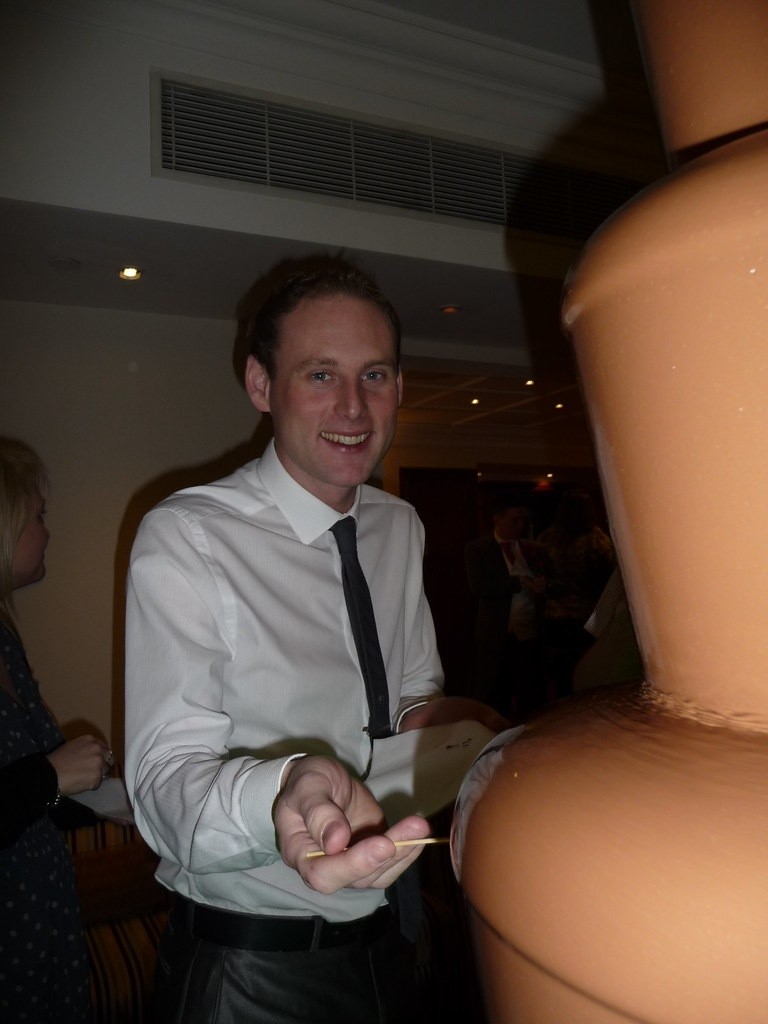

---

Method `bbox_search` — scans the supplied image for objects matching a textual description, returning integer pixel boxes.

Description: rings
[106,751,113,763]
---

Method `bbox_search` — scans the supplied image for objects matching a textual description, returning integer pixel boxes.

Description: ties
[331,516,395,739]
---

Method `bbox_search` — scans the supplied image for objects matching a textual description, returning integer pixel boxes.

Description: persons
[0,436,116,1024]
[122,252,515,1024]
[463,502,644,723]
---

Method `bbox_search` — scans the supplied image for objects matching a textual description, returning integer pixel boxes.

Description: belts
[192,896,469,952]
